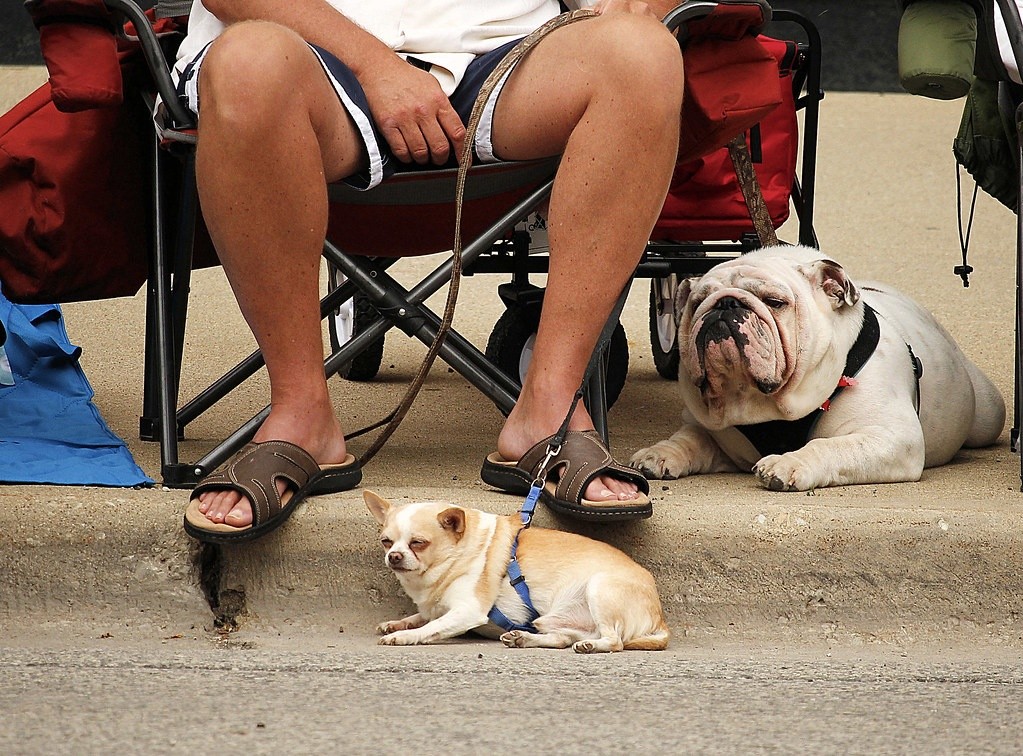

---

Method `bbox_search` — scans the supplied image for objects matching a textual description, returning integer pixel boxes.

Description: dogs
[629,245,1006,491]
[363,490,670,652]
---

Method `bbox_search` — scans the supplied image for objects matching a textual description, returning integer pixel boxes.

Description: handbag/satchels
[649,0,798,240]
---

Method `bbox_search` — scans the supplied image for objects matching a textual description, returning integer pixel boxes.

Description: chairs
[0,1,616,488]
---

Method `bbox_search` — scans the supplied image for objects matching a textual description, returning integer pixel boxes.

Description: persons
[172,0,684,546]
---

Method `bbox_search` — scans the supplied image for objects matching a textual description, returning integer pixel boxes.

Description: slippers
[480,429,652,522]
[184,440,363,544]
